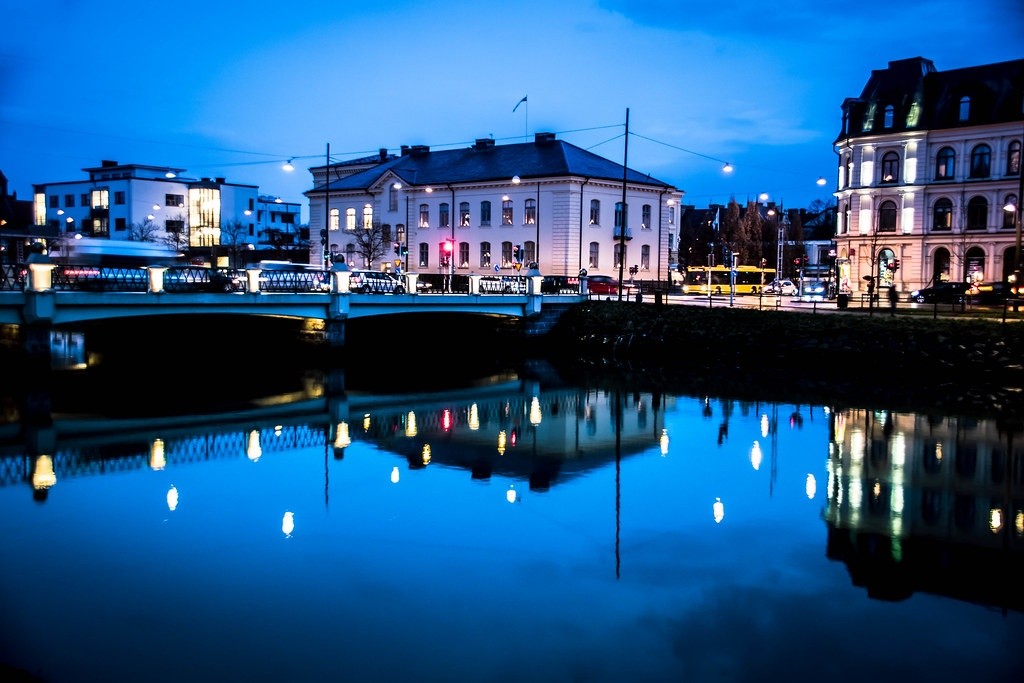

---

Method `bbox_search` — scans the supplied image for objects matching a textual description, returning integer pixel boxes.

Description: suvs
[350,269,406,294]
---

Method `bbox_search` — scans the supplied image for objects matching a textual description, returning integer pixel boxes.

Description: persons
[822,279,828,289]
[888,284,899,317]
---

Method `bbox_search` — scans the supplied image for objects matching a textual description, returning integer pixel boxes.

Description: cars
[588,275,639,297]
[761,279,797,295]
[806,282,833,296]
[910,281,1012,307]
[481,273,530,293]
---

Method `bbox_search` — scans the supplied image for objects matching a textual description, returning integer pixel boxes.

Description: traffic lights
[795,258,802,271]
[635,265,638,273]
[325,250,329,260]
[513,244,520,259]
[394,244,400,254]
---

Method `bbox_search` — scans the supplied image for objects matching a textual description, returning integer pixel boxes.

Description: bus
[684,266,775,295]
[55,240,326,296]
